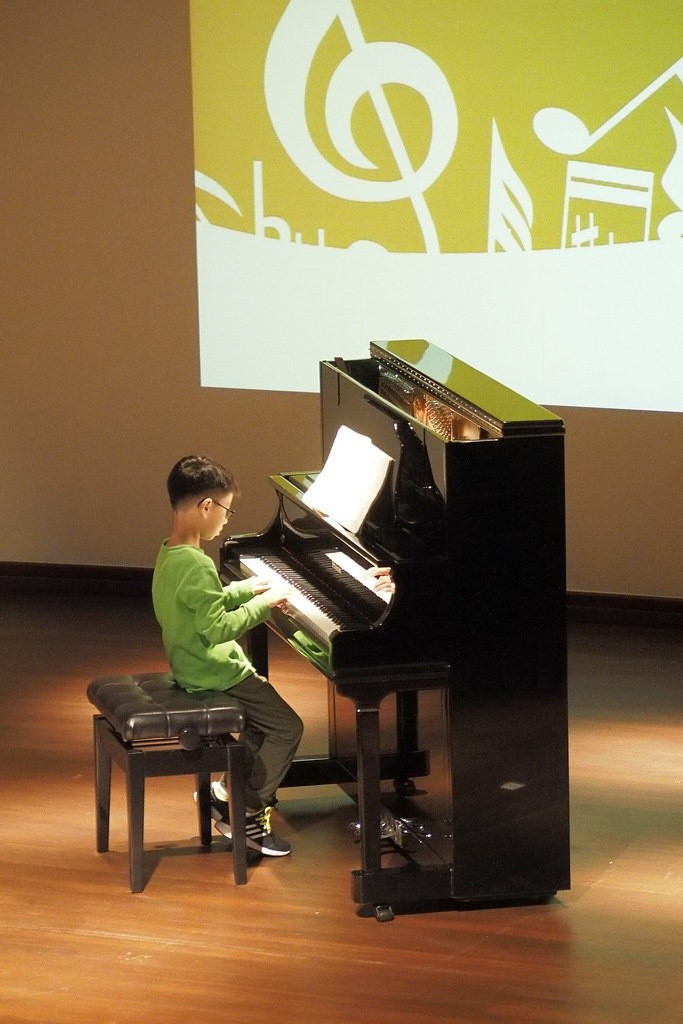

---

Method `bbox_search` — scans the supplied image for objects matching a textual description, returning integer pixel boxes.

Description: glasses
[196,496,235,520]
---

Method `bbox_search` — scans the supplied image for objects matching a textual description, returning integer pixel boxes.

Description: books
[302,425,396,533]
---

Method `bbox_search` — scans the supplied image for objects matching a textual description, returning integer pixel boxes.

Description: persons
[363,567,393,592]
[152,455,304,855]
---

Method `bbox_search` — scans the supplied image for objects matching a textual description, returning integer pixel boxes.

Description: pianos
[211,335,573,923]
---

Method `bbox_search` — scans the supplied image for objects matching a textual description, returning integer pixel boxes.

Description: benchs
[88,670,248,891]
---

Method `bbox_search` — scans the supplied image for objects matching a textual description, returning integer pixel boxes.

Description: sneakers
[193,774,229,821]
[214,799,291,857]
